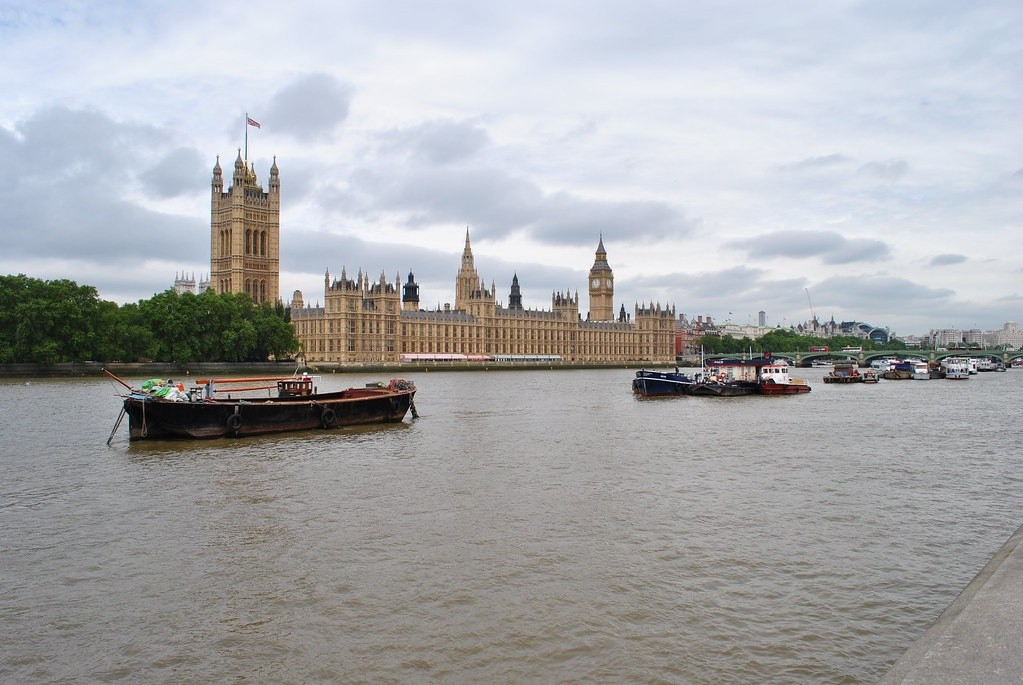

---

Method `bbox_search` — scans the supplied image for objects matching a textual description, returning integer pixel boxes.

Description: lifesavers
[322,408,335,424]
[227,414,243,430]
[721,372,726,378]
[392,401,400,414]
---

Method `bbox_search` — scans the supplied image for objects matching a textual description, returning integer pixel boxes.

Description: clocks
[592,278,600,289]
[606,279,613,289]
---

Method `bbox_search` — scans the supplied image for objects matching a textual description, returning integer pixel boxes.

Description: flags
[248,117,260,129]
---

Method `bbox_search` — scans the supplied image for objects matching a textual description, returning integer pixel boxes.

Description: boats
[874,356,931,380]
[632,367,694,395]
[101,366,416,446]
[939,356,1023,380]
[823,363,879,384]
[693,343,812,397]
